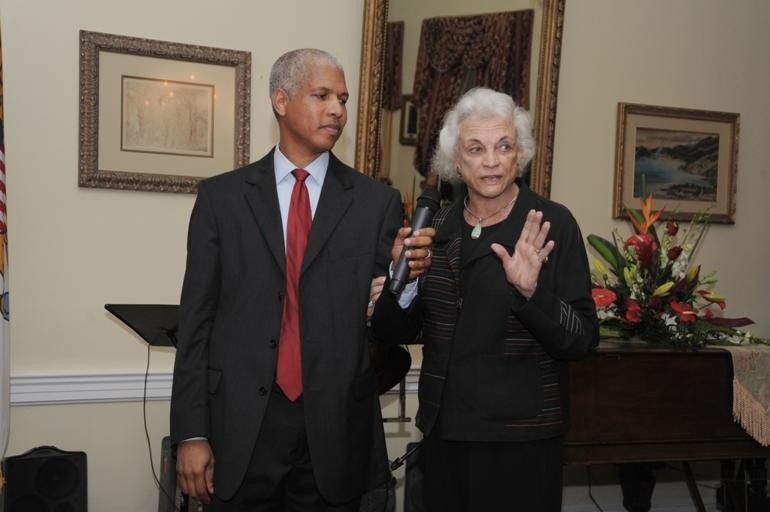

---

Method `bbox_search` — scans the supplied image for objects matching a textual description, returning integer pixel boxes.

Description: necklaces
[463,183,519,240]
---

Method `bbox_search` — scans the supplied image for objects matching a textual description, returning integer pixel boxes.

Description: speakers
[0,445,88,512]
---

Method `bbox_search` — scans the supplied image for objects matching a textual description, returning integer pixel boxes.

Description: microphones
[389,186,441,296]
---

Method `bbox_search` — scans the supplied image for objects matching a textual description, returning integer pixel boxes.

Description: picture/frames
[78,30,252,191]
[399,94,421,145]
[612,102,740,225]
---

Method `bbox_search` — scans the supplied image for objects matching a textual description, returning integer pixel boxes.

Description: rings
[368,299,376,307]
[425,249,430,259]
[534,246,543,253]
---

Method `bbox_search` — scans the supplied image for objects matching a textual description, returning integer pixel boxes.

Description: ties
[276,168,314,401]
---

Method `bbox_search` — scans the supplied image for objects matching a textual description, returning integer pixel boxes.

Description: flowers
[582,174,770,352]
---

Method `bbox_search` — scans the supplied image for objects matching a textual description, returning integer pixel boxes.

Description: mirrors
[354,1,564,227]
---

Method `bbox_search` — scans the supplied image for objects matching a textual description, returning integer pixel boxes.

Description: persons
[169,49,436,512]
[364,88,601,512]
